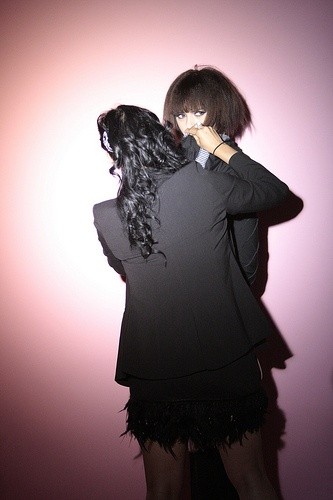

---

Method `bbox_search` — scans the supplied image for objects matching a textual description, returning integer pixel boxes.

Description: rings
[195,122,202,128]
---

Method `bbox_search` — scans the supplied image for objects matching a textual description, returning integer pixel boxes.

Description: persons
[163,64,261,500]
[93,104,294,500]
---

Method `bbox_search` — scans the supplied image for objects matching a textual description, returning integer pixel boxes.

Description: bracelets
[212,141,225,155]
